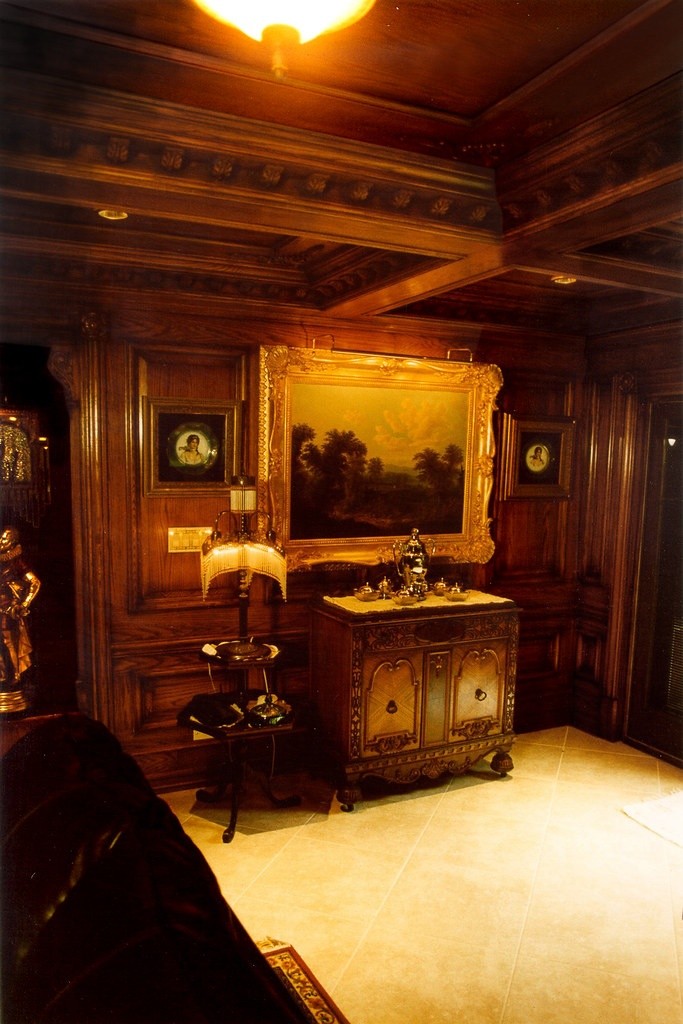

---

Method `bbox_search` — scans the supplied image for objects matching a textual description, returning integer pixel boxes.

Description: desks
[176,688,306,843]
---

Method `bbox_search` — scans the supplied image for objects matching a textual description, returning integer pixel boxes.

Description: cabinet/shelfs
[310,584,523,811]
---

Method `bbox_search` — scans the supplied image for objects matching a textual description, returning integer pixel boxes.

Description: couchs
[0,715,312,1024]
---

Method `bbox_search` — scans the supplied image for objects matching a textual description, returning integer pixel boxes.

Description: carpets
[255,934,354,1024]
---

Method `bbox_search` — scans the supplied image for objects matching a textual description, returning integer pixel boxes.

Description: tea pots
[443,583,470,601]
[408,583,428,600]
[390,585,417,604]
[430,578,452,596]
[355,583,380,602]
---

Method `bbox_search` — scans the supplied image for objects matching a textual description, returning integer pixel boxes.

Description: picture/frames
[140,393,249,499]
[258,342,506,574]
[498,410,579,503]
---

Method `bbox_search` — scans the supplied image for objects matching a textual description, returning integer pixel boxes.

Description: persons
[530,447,545,467]
[0,525,40,686]
[179,435,205,463]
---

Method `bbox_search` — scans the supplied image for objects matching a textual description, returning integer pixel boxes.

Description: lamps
[201,473,288,662]
[97,207,132,222]
[549,274,577,285]
